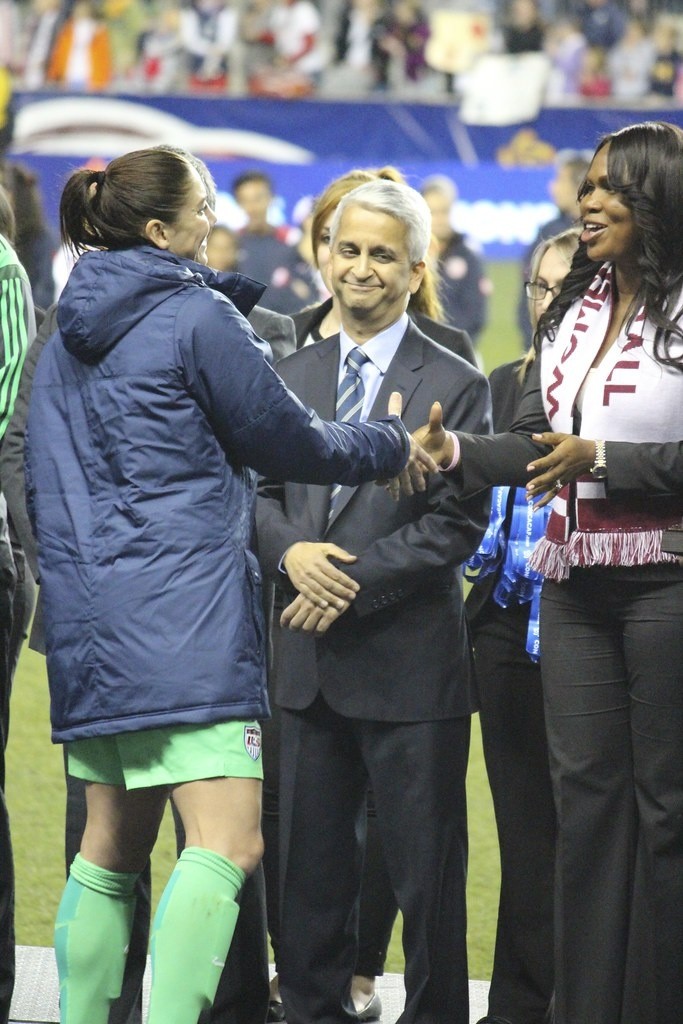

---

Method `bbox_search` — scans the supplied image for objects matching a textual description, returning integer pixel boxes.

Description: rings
[556,478,563,490]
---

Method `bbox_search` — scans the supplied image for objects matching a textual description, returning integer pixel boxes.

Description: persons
[0,147,277,1024]
[3,143,440,1023]
[419,172,487,346]
[514,147,599,354]
[0,0,683,320]
[461,225,593,1024]
[243,163,493,1023]
[246,176,481,1019]
[395,122,683,1024]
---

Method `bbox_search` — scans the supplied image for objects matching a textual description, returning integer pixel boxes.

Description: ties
[328,347,368,521]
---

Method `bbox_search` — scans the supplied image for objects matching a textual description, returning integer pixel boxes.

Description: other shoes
[357,993,381,1020]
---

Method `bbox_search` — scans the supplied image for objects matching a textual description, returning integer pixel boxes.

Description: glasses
[523,282,562,300]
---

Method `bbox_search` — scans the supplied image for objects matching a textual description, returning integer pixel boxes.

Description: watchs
[589,439,607,482]
